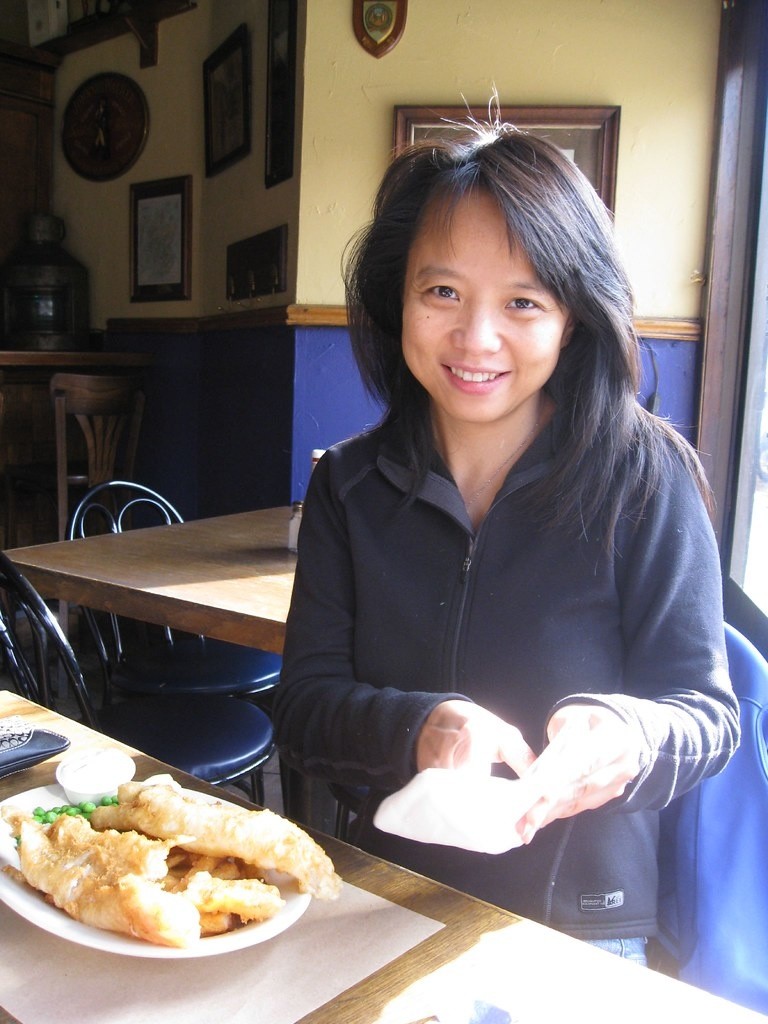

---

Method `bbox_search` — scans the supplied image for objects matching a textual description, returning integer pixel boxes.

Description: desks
[2,502,303,657]
[0,350,162,464]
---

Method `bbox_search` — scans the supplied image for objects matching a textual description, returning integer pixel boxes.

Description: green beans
[30,793,118,825]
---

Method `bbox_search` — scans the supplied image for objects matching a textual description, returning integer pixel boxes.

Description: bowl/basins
[55,747,137,808]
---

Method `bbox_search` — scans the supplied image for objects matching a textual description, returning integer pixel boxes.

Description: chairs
[335,576,768,1019]
[63,479,290,816]
[0,552,277,808]
[4,371,150,646]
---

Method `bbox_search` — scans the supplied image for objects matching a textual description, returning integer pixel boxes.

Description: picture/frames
[265,0,295,191]
[204,23,252,180]
[393,102,623,219]
[129,174,193,301]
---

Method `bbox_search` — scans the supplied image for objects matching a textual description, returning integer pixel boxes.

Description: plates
[0,784,312,959]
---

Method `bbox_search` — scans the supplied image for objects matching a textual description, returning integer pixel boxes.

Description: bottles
[310,449,327,480]
[288,501,303,553]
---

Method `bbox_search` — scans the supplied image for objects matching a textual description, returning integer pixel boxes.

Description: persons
[275,134,742,969]
[88,93,110,160]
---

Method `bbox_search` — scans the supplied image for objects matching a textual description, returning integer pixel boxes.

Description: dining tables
[0,691,768,1024]
